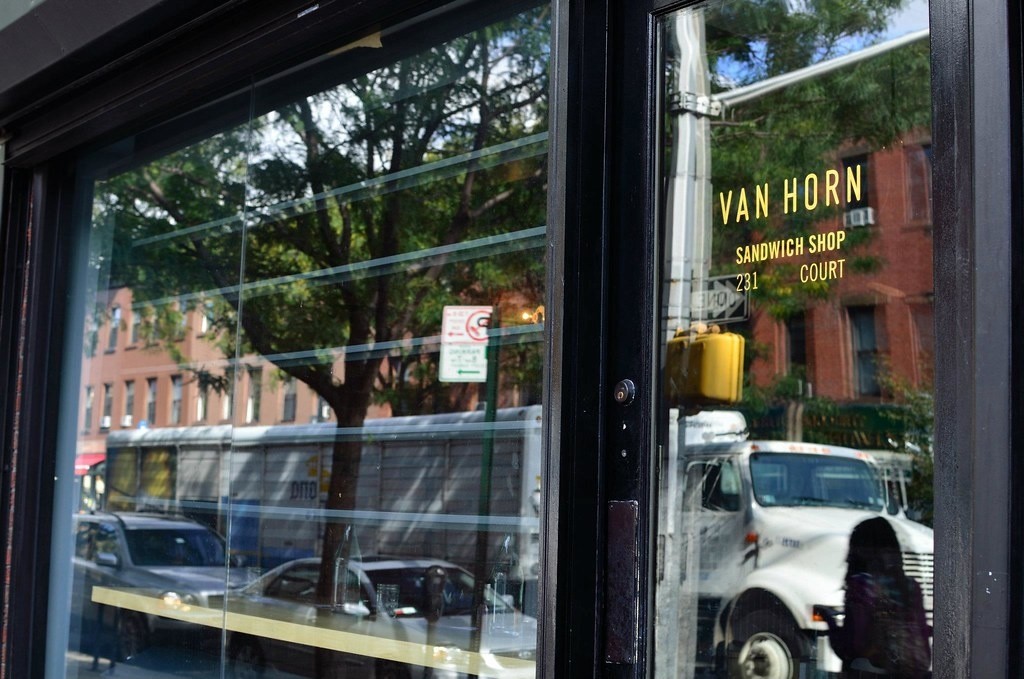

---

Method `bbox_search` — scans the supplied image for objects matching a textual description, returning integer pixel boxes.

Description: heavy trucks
[80,404,934,679]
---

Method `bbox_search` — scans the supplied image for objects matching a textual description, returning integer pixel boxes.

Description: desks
[91,585,537,679]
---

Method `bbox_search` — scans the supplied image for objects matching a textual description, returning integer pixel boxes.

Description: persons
[811,515,932,678]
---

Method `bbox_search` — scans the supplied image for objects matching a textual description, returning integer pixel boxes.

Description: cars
[219,554,539,679]
[70,510,314,664]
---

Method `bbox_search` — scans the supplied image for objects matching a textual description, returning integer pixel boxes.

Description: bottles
[489,537,524,636]
[331,525,362,613]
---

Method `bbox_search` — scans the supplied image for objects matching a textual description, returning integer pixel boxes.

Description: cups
[377,584,398,620]
